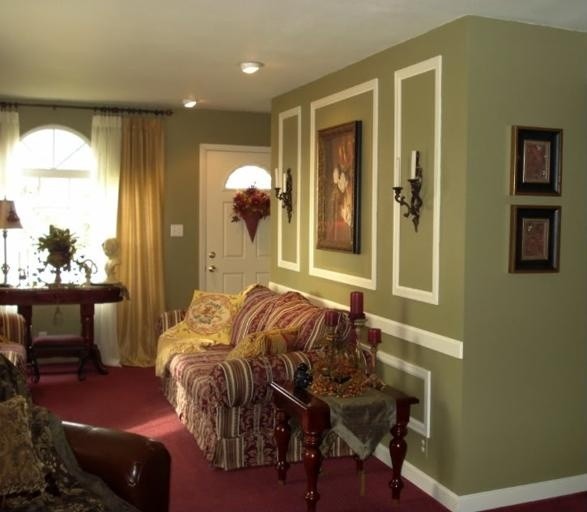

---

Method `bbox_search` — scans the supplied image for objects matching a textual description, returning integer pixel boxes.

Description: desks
[1,283,123,376]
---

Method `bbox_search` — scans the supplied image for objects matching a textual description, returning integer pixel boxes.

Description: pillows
[178,289,245,347]
[1,393,46,499]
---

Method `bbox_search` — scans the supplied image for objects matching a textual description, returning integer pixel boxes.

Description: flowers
[229,182,271,224]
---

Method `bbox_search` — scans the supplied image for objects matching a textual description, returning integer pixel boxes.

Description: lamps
[0,196,24,287]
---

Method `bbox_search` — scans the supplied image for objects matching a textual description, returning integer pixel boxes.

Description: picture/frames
[507,124,563,275]
[315,120,362,255]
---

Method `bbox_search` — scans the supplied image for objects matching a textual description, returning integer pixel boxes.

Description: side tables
[268,376,420,512]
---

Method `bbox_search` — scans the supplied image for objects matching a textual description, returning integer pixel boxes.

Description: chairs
[62,420,171,511]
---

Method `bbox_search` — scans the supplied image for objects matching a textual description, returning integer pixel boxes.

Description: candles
[324,291,381,345]
[395,150,417,188]
[275,168,287,193]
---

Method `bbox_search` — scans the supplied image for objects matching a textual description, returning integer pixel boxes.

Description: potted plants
[33,224,80,271]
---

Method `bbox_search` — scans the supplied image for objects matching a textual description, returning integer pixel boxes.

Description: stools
[29,334,91,383]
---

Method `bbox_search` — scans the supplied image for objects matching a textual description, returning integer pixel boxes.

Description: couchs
[154,283,359,471]
[0,309,27,374]
